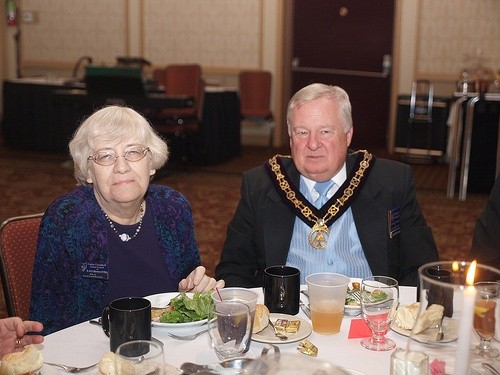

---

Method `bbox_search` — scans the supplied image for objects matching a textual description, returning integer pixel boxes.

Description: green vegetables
[345,287,387,305]
[160,288,215,323]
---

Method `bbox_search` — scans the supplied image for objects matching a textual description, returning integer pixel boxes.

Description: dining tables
[35,285,500,375]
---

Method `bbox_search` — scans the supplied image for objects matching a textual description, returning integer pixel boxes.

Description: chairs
[84,75,139,110]
[238,70,275,149]
[151,64,205,178]
[0,213,44,320]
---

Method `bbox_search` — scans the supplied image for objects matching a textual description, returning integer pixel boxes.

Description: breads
[98,351,137,375]
[234,304,270,333]
[0,345,44,375]
[396,302,444,333]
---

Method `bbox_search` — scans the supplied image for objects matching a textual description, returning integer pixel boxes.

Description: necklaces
[100,205,143,242]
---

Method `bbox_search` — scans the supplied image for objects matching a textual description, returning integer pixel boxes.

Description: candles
[453,260,478,375]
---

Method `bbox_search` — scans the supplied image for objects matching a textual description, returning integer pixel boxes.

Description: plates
[250,313,312,344]
[390,324,458,344]
[143,291,196,309]
[344,277,393,316]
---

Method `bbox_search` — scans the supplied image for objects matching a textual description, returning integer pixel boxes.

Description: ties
[313,180,334,210]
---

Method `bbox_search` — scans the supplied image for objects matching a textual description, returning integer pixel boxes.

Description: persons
[216,83,441,309]
[462,174,500,287]
[0,317,44,375]
[27,105,226,339]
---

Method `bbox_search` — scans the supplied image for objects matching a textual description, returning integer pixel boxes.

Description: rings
[16,338,21,348]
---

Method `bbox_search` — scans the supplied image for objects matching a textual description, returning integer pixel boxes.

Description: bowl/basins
[418,260,500,322]
[151,311,217,340]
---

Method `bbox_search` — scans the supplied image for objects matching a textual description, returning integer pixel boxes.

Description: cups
[361,276,399,351]
[207,302,256,357]
[306,272,351,335]
[101,296,152,357]
[473,282,500,358]
[261,265,301,315]
[212,287,258,307]
[116,340,166,375]
[416,267,454,317]
[389,348,429,375]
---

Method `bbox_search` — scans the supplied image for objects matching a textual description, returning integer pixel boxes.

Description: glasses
[88,147,149,166]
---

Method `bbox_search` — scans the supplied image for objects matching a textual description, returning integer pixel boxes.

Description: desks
[4,77,241,168]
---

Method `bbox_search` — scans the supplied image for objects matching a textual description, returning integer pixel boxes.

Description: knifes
[299,299,310,318]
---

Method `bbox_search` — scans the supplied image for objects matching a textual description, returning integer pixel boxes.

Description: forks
[167,326,215,342]
[44,362,99,374]
